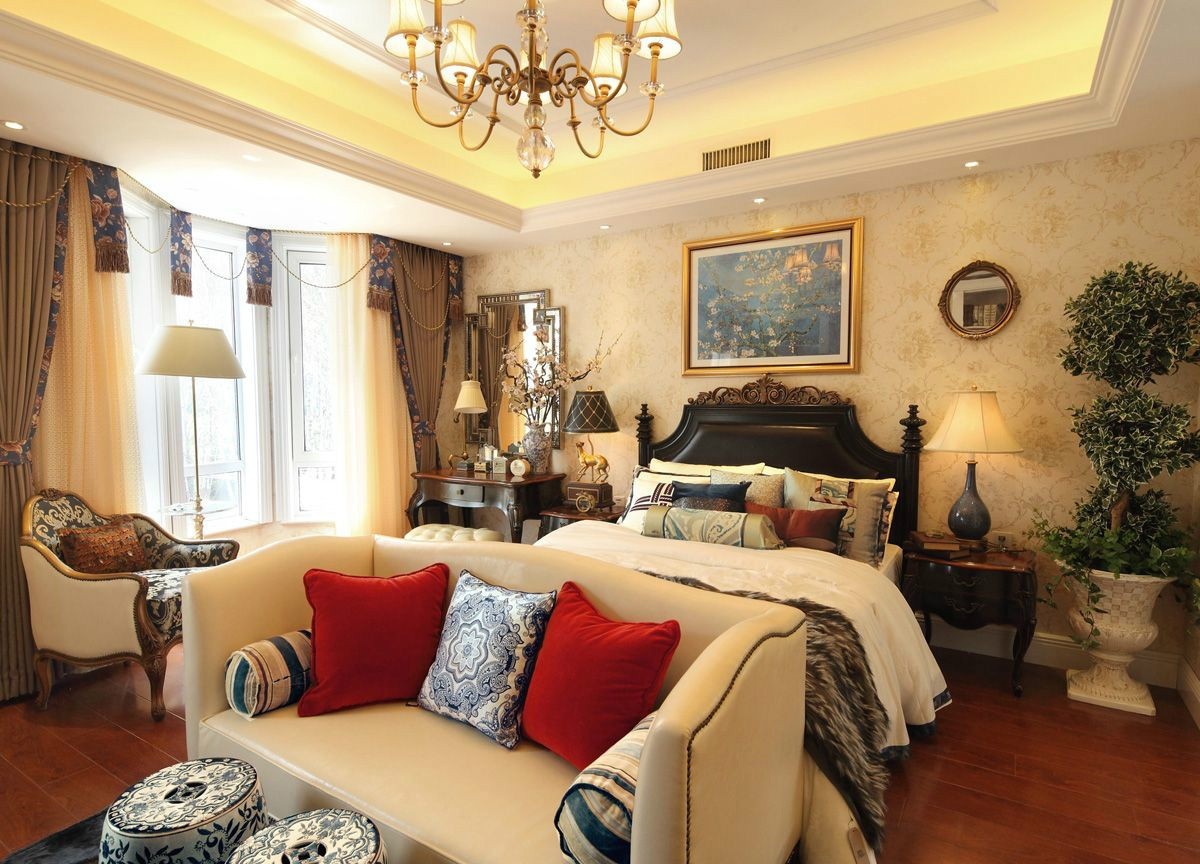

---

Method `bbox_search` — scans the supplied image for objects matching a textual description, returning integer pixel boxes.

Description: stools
[225,807,390,863]
[97,755,267,863]
[404,523,505,544]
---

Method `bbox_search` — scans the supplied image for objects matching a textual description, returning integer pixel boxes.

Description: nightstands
[904,548,1039,699]
[536,505,628,542]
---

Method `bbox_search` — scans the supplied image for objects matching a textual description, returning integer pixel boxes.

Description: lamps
[447,379,488,468]
[141,319,247,538]
[383,0,684,180]
[918,385,1025,554]
[561,386,621,513]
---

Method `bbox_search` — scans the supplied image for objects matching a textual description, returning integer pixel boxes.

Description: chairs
[18,488,239,725]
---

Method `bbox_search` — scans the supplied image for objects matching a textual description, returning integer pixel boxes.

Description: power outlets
[992,531,1014,551]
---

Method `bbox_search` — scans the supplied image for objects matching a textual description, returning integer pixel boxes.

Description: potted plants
[1020,262,1200,718]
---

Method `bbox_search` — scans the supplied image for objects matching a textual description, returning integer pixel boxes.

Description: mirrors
[937,260,1021,341]
[463,288,568,458]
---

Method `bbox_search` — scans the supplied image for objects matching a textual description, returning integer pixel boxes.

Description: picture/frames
[681,216,865,375]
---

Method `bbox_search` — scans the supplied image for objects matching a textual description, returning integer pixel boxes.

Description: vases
[520,421,555,475]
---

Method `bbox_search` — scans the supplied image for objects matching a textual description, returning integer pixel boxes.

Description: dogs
[575,441,610,483]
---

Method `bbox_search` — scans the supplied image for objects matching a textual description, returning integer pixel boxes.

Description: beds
[535,374,953,863]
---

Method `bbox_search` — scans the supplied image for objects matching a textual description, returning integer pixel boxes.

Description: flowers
[494,319,625,424]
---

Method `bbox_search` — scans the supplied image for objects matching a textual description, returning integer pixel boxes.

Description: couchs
[182,531,809,864]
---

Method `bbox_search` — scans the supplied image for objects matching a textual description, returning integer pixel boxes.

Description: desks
[404,467,567,543]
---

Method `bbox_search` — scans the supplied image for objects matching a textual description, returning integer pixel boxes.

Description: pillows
[554,707,662,864]
[417,567,557,750]
[671,480,753,513]
[709,469,785,508]
[625,466,710,510]
[744,501,848,552]
[523,581,683,772]
[54,518,147,572]
[622,476,673,532]
[649,458,765,478]
[877,491,899,563]
[224,626,314,721]
[640,506,785,550]
[784,466,895,571]
[297,561,453,717]
